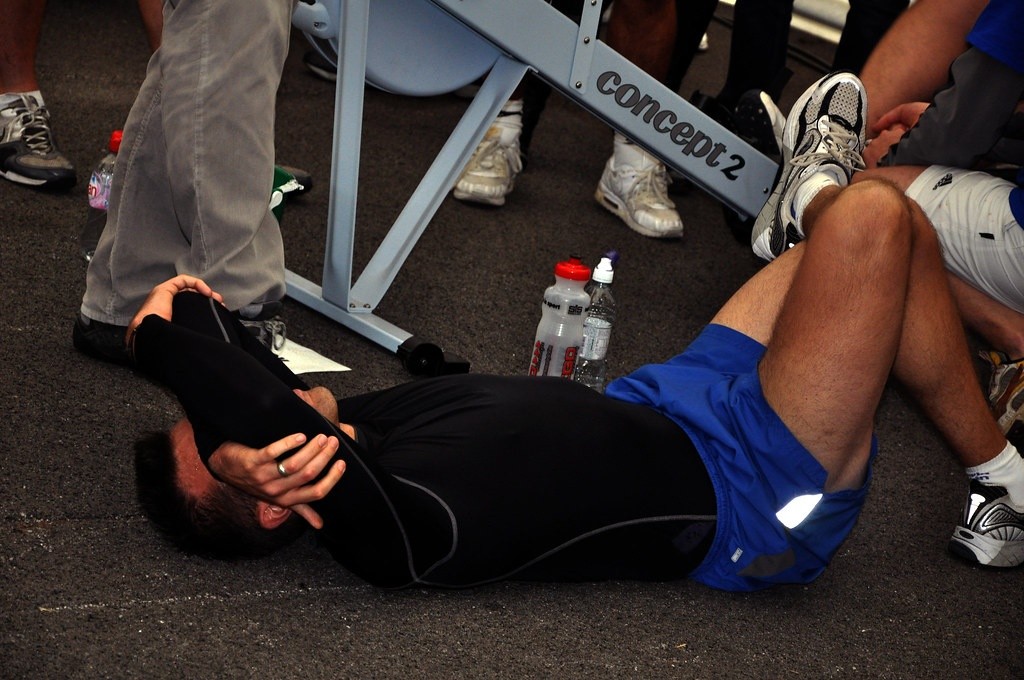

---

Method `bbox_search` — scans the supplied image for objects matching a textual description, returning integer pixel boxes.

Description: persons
[124,69,1024,588]
[449,0,1024,438]
[0,0,313,392]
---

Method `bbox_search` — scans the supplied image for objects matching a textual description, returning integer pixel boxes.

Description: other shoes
[238,298,287,355]
[73,311,135,367]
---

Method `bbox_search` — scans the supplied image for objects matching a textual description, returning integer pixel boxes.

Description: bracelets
[128,328,136,358]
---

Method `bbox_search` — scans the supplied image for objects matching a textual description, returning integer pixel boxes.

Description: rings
[278,463,288,476]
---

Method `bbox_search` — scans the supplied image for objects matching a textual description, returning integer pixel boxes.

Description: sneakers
[750,72,869,262]
[0,93,74,185]
[978,346,1023,441]
[949,478,1024,568]
[454,124,523,205]
[594,155,683,236]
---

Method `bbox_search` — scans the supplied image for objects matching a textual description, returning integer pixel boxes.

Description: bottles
[77,129,123,261]
[527,259,591,380]
[568,258,616,396]
